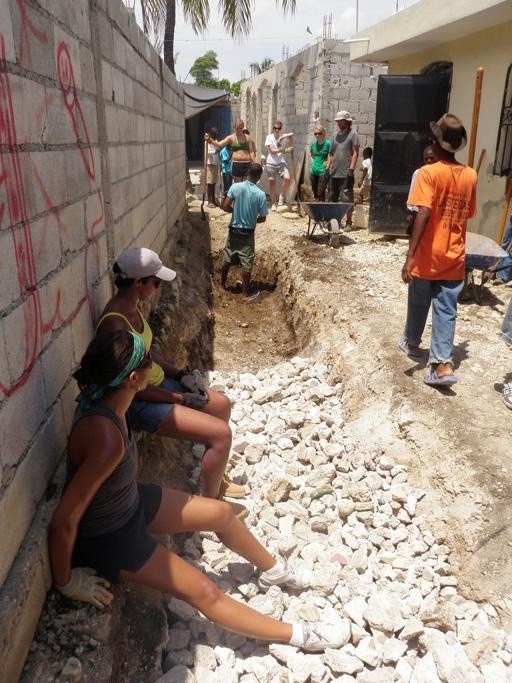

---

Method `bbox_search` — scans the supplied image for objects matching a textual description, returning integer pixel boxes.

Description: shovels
[200,139,212,222]
[288,135,305,218]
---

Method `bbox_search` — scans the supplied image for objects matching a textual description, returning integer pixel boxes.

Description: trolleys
[298,198,355,248]
[458,231,509,304]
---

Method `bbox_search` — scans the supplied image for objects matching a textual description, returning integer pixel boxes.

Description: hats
[334,110,352,122]
[112,246,177,282]
[428,110,468,154]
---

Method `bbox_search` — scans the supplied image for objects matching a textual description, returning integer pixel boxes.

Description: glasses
[274,127,281,130]
[136,351,153,370]
[314,132,324,135]
[143,279,161,289]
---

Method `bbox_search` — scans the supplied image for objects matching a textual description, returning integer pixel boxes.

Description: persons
[500,297,512,409]
[47,329,354,653]
[220,162,269,302]
[399,111,479,388]
[92,246,250,521]
[203,109,373,211]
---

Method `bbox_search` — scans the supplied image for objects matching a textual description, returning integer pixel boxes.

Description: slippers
[423,369,458,386]
[399,338,422,357]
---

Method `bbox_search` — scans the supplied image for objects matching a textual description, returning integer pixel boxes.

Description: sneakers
[199,472,246,499]
[302,617,352,653]
[217,496,250,522]
[256,555,313,590]
[502,381,512,409]
[271,203,277,211]
[207,201,219,208]
[278,193,285,206]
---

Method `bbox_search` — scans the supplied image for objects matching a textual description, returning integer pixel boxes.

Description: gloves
[175,368,209,400]
[55,566,114,610]
[173,393,207,411]
